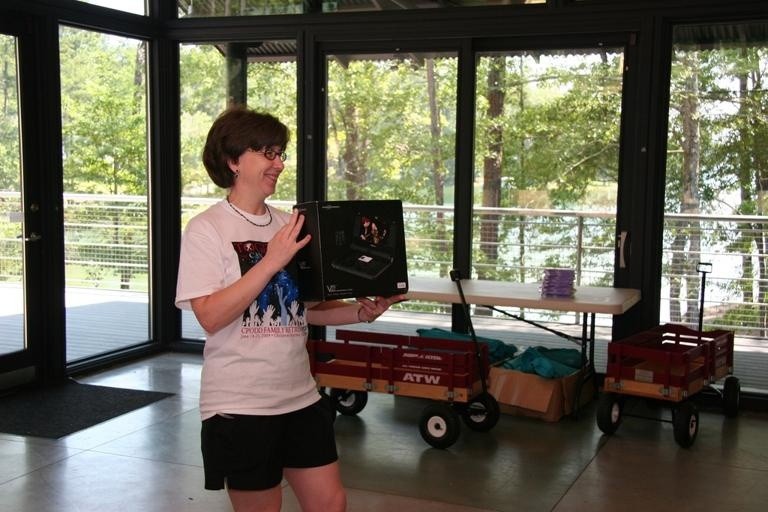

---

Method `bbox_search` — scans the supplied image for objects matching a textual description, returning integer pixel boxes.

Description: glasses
[264,150,286,162]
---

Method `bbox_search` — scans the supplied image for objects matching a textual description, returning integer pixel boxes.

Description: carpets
[0,379,177,440]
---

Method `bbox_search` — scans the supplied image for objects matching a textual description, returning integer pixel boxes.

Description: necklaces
[226,192,273,226]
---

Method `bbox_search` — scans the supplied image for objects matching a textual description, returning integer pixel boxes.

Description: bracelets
[358,308,374,324]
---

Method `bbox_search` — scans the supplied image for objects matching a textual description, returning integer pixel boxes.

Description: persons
[175,107,407,512]
[359,216,373,244]
[242,285,304,326]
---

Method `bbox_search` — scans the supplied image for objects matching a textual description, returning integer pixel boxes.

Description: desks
[401,277,640,422]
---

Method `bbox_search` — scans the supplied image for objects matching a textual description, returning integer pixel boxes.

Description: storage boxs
[487,352,594,423]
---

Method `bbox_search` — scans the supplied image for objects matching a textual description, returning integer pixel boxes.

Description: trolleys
[597,260,741,448]
[307,269,499,450]
